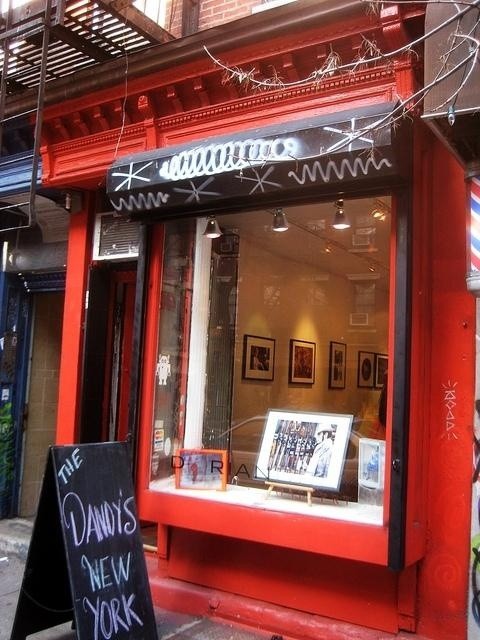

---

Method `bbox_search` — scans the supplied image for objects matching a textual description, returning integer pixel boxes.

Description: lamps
[203,198,349,239]
[370,200,390,222]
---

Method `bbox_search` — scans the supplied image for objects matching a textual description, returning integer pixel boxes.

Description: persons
[191,457,199,485]
[305,423,335,479]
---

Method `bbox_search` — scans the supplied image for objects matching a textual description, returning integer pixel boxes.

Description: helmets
[316,423,333,433]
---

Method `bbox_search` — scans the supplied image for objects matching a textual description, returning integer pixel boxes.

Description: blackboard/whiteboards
[51,441,158,640]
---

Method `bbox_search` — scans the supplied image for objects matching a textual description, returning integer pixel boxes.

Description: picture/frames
[241,333,388,391]
[174,407,385,497]
